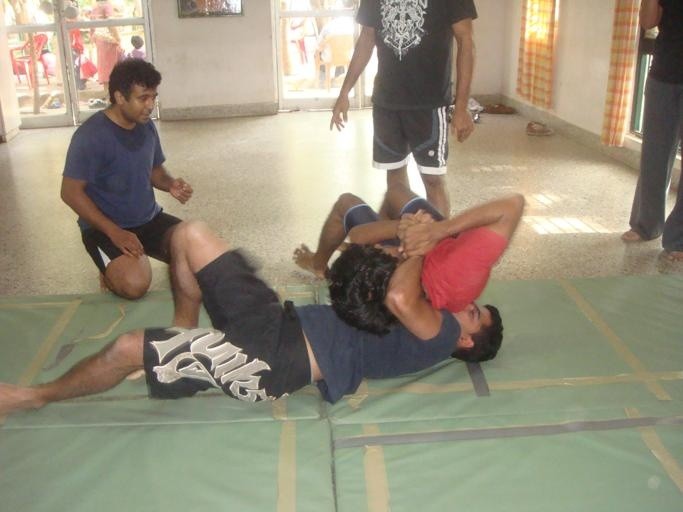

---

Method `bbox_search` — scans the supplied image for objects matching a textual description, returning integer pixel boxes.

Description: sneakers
[88,99,105,108]
[526,121,554,136]
[485,104,514,113]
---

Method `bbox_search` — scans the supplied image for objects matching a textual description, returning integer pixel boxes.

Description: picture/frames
[178,0,244,19]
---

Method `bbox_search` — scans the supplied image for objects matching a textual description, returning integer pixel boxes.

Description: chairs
[10,34,49,89]
[314,35,355,91]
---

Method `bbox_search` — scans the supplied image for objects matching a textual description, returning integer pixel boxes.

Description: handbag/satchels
[93,19,118,44]
[80,59,98,80]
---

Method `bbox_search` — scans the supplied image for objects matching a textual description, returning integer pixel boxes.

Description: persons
[59,58,194,301]
[289,0,364,80]
[330,1,478,217]
[292,181,524,335]
[2,0,148,105]
[0,218,506,417]
[621,0,683,259]
